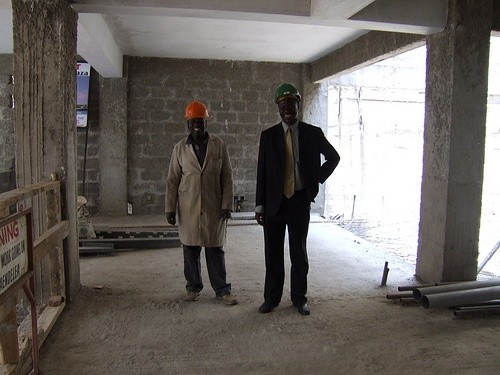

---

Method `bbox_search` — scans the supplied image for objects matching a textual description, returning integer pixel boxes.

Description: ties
[284,127,295,200]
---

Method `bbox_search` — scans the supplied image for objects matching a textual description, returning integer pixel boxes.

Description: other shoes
[217,294,238,305]
[183,290,201,301]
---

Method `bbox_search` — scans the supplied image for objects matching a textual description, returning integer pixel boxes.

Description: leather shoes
[258,302,279,313]
[293,303,310,315]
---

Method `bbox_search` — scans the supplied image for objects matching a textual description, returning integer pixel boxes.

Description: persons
[165,101,239,306]
[255,83,340,315]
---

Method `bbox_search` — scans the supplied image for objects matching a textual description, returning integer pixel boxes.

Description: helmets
[186,101,209,119]
[274,82,301,102]
